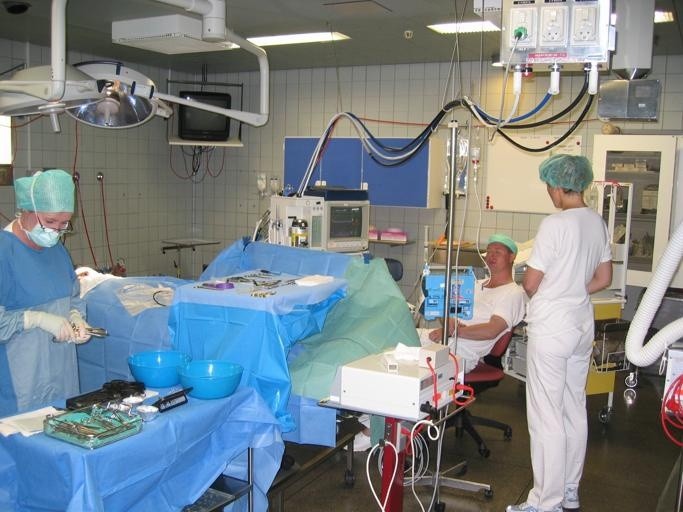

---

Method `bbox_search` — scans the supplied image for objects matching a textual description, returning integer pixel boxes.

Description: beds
[69,266,368,486]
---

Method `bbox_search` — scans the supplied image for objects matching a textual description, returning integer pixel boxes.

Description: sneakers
[562,489,580,509]
[505,500,563,512]
[342,431,372,452]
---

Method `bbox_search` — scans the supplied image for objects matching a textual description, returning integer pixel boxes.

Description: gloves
[23,310,74,342]
[70,310,92,345]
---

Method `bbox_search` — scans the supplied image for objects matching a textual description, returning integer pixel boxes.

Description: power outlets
[540,6,569,47]
[510,7,537,48]
[572,5,599,46]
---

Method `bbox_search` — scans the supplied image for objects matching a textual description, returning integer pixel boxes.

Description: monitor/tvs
[178,90,233,142]
[324,200,370,254]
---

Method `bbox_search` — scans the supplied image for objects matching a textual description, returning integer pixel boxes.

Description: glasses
[37,214,74,234]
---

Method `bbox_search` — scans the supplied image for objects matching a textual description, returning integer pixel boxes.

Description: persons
[0,169,90,414]
[505,154,615,512]
[416,234,527,377]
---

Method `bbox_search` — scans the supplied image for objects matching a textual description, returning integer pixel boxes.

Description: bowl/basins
[137,405,159,422]
[127,349,194,388]
[122,397,144,407]
[178,361,244,399]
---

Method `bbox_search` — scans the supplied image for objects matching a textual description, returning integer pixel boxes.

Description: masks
[17,218,69,248]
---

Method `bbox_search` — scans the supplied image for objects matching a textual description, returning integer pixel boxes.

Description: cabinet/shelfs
[593,136,675,289]
[502,297,623,425]
[0,377,285,510]
[284,136,444,211]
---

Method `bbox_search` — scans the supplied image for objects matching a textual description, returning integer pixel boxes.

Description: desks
[316,395,492,512]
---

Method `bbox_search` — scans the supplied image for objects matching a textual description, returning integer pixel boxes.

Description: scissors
[52,321,107,343]
[45,400,131,440]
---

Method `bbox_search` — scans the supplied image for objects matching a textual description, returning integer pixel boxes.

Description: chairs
[417,326,516,458]
